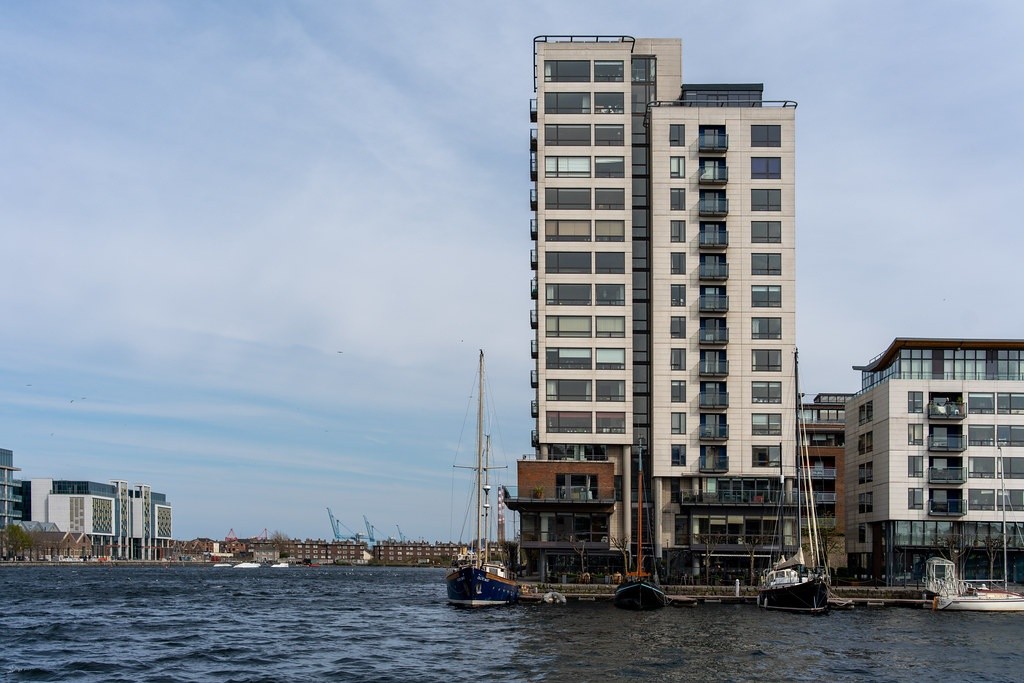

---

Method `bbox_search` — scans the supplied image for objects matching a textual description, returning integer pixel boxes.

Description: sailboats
[612,434,667,611]
[922,444,1024,612]
[753,346,834,616]
[440,349,523,609]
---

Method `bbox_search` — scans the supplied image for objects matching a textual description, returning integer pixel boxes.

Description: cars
[0,554,128,563]
